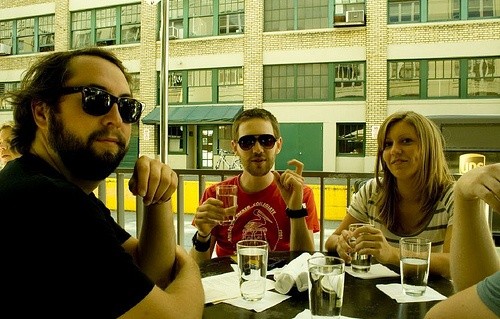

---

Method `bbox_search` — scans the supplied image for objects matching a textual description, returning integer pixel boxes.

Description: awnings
[142,104,243,124]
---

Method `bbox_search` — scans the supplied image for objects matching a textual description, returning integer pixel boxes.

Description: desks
[163,250,455,319]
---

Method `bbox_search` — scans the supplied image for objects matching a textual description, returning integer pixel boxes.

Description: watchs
[285,203,308,219]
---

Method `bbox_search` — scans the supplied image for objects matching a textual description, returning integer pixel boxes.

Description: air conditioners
[169,26,179,40]
[0,43,12,56]
[346,10,364,23]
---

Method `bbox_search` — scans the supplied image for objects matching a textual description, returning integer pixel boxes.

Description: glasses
[60,85,142,123]
[236,134,277,151]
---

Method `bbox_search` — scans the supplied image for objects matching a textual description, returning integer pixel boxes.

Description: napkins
[201,271,276,303]
[222,290,292,313]
[291,308,360,319]
[376,283,448,304]
[294,251,327,292]
[273,251,312,295]
[230,264,276,276]
[345,263,400,279]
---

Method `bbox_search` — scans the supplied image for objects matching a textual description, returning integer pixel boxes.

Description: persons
[0,48,205,319]
[0,120,22,165]
[189,109,320,264]
[325,111,457,280]
[424,163,500,319]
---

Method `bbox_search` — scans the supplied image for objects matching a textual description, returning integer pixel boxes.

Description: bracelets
[198,232,211,238]
[192,231,211,252]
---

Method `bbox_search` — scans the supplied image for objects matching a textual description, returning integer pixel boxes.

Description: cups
[307,256,345,319]
[399,237,432,298]
[216,184,238,227]
[348,223,375,274]
[236,239,269,302]
